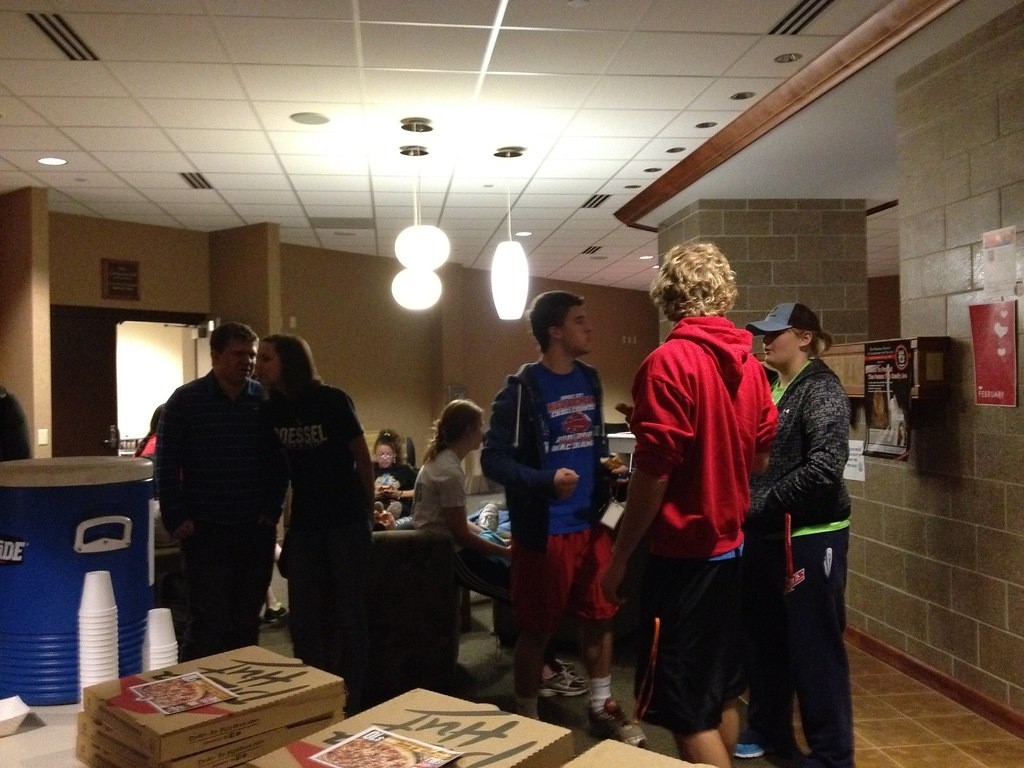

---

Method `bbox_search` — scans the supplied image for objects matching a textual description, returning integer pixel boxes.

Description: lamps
[485,146,531,323]
[392,144,442,312]
[395,114,453,269]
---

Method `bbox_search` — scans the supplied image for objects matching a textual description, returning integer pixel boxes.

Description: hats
[745,302,821,336]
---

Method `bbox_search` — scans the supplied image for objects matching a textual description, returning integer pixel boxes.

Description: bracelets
[397,490,403,500]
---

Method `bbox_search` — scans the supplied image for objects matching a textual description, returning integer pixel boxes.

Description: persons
[597,238,777,768]
[481,290,647,748]
[0,321,628,714]
[735,303,858,768]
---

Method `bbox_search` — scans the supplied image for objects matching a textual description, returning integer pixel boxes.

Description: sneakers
[734,735,797,758]
[587,696,648,748]
[538,651,588,698]
[259,604,288,629]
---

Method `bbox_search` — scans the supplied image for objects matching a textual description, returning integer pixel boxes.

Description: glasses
[376,452,395,459]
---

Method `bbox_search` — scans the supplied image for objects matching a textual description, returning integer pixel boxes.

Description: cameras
[599,501,624,528]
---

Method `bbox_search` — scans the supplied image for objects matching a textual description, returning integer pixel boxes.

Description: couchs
[362,499,473,692]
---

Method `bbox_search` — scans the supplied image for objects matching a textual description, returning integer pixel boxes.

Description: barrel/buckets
[0,454,159,708]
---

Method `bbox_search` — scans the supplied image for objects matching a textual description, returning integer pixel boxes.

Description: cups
[141,607,180,674]
[76,569,121,711]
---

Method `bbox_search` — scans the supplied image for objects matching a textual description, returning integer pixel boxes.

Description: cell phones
[379,486,391,490]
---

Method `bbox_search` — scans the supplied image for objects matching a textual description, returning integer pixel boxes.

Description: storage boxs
[76,646,721,768]
